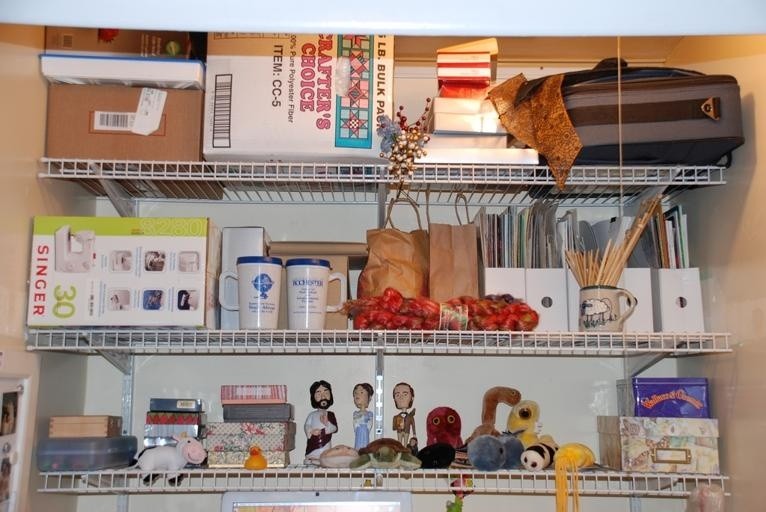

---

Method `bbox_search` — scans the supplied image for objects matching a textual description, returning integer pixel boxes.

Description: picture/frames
[0,376,33,512]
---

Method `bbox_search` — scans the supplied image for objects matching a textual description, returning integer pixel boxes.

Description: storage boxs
[44,82,224,202]
[421,93,517,135]
[473,261,708,349]
[48,414,123,439]
[614,376,711,420]
[267,254,352,345]
[201,32,396,195]
[35,435,138,471]
[594,415,724,478]
[203,418,296,453]
[219,225,271,341]
[27,213,221,346]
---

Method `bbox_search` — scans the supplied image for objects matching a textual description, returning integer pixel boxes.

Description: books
[480,199,690,269]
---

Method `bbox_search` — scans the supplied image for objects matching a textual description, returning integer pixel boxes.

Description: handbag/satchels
[422,187,484,303]
[354,198,430,303]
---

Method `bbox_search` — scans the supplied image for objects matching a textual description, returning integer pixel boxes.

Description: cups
[576,285,638,335]
[286,255,346,336]
[217,253,283,334]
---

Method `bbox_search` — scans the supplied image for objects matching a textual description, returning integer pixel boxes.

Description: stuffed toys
[319,386,595,512]
[125,431,207,487]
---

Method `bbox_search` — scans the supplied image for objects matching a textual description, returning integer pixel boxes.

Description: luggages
[536,53,751,169]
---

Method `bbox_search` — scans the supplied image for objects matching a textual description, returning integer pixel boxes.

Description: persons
[304,381,338,459]
[352,383,373,450]
[393,383,416,449]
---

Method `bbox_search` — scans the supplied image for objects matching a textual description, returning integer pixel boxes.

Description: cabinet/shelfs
[30,157,734,498]
